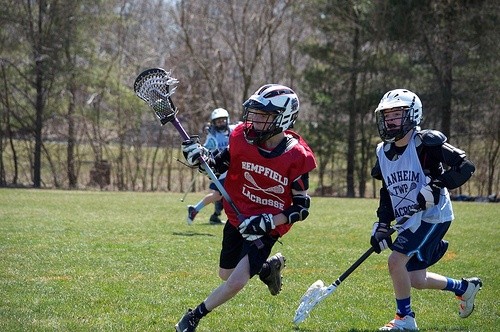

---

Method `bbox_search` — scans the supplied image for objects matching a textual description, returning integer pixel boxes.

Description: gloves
[182,135,216,175]
[370,222,393,254]
[239,213,276,241]
[417,176,443,210]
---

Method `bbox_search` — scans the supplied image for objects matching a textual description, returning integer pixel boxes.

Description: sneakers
[175,308,200,332]
[210,215,226,224]
[259,253,286,296]
[379,312,419,332]
[187,205,199,224]
[454,277,482,318]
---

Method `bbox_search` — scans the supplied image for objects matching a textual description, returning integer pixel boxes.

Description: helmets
[375,89,423,144]
[211,108,229,122]
[242,84,300,146]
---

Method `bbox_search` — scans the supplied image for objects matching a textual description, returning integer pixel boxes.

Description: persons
[175,83,316,332]
[188,107,244,224]
[369,88,482,331]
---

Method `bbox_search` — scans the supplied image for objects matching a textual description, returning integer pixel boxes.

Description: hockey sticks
[180,170,199,202]
[133,68,265,249]
[291,204,422,326]
